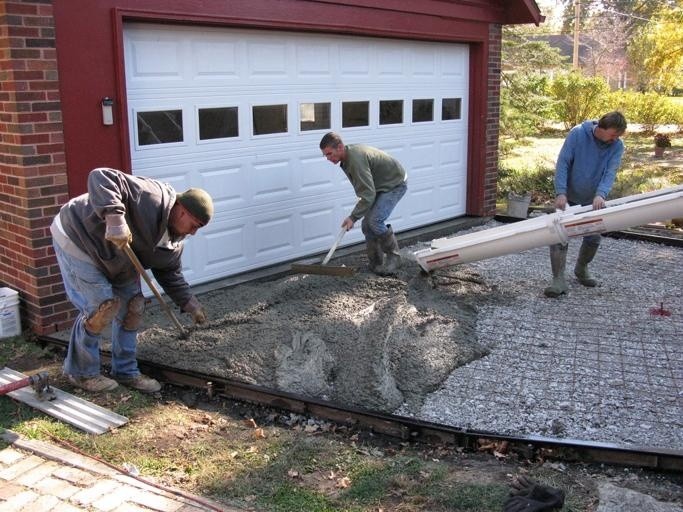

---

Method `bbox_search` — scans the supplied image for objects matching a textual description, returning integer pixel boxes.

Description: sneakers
[68,372,118,392]
[109,372,162,392]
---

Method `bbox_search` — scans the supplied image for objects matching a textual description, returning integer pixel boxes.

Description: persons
[543,111,629,295]
[320,132,409,275]
[48,167,216,396]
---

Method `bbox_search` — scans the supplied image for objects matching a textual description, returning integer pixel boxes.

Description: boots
[365,223,403,275]
[573,239,600,287]
[543,242,569,298]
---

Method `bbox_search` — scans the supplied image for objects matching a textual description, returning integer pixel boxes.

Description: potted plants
[654,134,671,157]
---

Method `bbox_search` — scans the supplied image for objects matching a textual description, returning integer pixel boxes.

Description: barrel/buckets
[0,287,22,338]
[507,195,532,220]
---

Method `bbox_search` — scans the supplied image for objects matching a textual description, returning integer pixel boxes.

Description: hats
[175,186,214,225]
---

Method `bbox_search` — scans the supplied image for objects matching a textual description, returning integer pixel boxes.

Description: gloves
[182,293,208,325]
[104,222,133,251]
